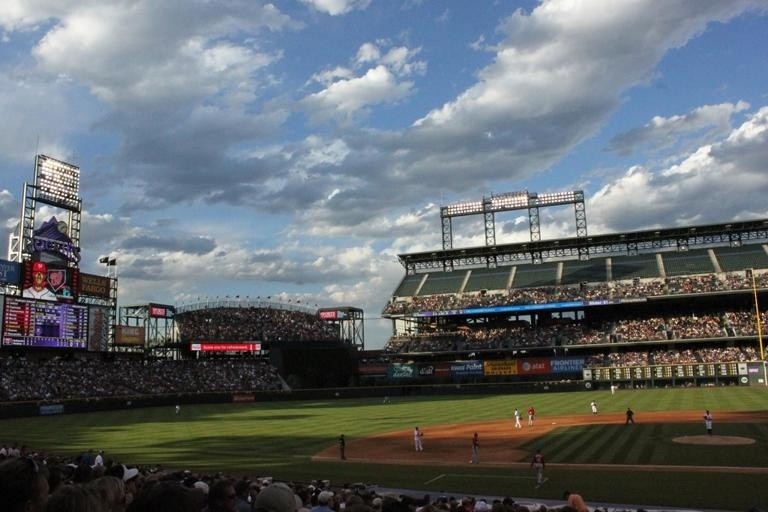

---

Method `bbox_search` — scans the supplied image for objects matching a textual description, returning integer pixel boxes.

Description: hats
[254,481,302,511]
[122,463,138,484]
[318,491,334,504]
[372,498,381,508]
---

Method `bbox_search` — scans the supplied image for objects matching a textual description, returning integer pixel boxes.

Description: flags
[106,259,116,266]
[99,256,109,263]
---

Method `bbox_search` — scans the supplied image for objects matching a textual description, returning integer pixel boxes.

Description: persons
[512,408,522,428]
[0,442,645,512]
[703,409,713,436]
[624,407,636,425]
[384,268,767,379]
[611,382,618,399]
[527,405,535,427]
[0,299,341,404]
[468,432,480,464]
[590,399,598,416]
[21,261,59,303]
[413,426,425,451]
[338,433,347,460]
